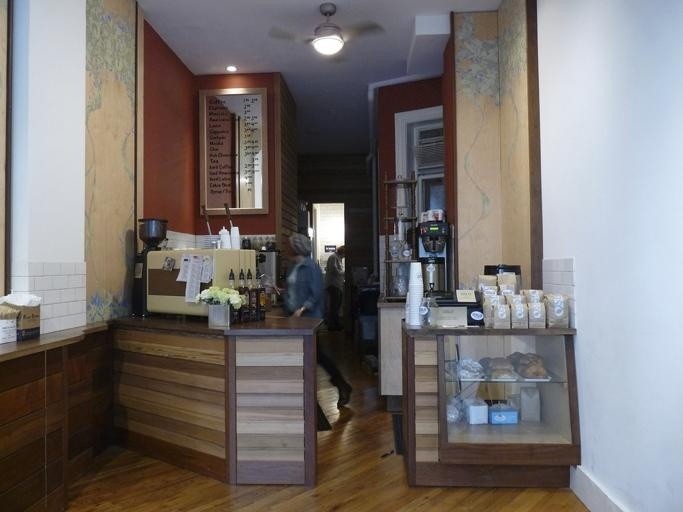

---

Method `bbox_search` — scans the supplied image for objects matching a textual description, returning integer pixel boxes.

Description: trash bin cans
[393,414,405,455]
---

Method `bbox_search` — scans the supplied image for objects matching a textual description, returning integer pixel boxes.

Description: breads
[479,352,548,380]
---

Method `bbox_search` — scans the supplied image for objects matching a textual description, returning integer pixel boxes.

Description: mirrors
[198,86,269,217]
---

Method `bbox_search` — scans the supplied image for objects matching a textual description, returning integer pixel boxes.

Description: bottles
[242,235,275,251]
[228,269,266,322]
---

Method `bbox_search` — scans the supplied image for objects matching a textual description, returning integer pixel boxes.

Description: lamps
[309,2,345,56]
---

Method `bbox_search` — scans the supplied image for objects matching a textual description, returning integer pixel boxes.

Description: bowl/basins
[427,209,445,222]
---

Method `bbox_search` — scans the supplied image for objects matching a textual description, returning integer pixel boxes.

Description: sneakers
[337,383,352,407]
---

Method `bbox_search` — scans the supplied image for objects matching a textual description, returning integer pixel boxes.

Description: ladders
[383,168,417,302]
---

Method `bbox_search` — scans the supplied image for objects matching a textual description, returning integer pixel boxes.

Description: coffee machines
[134,218,170,317]
[416,221,452,300]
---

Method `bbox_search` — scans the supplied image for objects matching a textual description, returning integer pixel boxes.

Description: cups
[403,261,423,327]
[389,240,412,261]
[270,292,276,303]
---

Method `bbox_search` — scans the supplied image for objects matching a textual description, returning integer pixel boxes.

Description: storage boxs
[461,397,490,426]
[0,299,42,343]
[0,306,21,345]
[487,405,518,426]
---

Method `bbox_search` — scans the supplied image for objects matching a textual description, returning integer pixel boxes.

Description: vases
[207,303,233,331]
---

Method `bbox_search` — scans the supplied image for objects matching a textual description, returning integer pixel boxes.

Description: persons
[265,232,352,406]
[324,246,346,331]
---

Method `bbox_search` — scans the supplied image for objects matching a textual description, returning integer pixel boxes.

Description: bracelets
[299,306,304,313]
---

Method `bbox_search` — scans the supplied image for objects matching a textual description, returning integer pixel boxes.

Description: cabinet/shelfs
[396,316,584,491]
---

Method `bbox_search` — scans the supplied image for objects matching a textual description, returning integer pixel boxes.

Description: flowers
[195,285,246,310]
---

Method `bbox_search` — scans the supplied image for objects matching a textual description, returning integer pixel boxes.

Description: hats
[288,233,312,258]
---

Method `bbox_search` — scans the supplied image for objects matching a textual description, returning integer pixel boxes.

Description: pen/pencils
[381,450,394,457]
